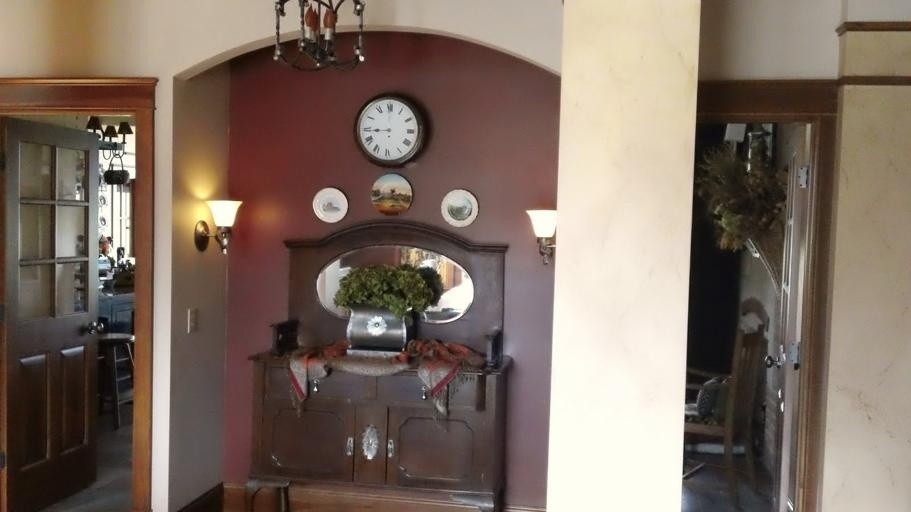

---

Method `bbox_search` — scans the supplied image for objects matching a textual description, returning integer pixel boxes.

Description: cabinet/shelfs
[246,217,512,511]
[109,302,135,394]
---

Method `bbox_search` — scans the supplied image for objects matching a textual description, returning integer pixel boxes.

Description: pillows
[695,374,731,423]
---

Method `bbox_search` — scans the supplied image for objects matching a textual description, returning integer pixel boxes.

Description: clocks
[353,94,427,169]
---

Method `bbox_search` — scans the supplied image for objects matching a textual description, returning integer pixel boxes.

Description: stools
[97,333,135,432]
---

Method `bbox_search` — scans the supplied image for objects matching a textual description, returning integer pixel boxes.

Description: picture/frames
[440,188,479,228]
[312,187,349,224]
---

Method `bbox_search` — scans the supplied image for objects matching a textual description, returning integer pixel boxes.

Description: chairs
[681,324,767,509]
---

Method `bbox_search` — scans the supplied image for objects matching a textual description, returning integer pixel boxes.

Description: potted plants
[695,142,785,254]
[332,263,436,359]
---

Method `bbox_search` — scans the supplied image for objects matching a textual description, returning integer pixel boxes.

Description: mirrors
[316,243,476,326]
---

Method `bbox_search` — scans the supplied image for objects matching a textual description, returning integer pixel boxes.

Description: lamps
[85,115,134,160]
[524,208,555,266]
[271,0,367,72]
[194,200,244,256]
